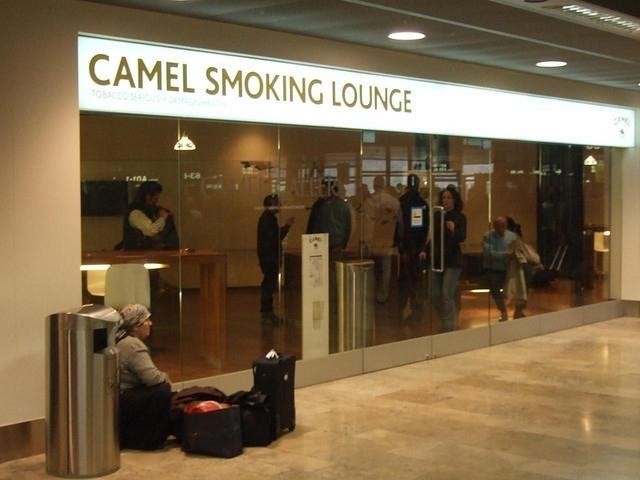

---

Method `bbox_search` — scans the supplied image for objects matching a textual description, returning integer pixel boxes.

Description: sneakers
[514,311,526,319]
[497,312,508,321]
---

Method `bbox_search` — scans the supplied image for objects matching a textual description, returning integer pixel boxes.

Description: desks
[81,246,231,372]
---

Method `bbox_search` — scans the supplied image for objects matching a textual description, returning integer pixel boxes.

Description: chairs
[104,261,152,316]
[85,269,108,298]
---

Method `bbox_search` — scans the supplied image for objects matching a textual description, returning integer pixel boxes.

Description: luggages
[252,350,298,433]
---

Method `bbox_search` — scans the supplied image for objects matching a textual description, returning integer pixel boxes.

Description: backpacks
[118,387,165,452]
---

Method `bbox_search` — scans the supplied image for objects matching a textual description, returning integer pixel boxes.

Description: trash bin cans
[329,258,376,355]
[45,303,125,478]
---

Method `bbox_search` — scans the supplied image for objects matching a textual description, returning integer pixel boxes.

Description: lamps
[174,117,197,155]
[583,146,598,167]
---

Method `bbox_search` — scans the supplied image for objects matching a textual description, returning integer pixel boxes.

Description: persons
[305,176,352,262]
[393,174,430,323]
[257,193,295,325]
[122,181,172,307]
[419,187,467,333]
[481,217,526,321]
[357,174,405,324]
[115,304,175,451]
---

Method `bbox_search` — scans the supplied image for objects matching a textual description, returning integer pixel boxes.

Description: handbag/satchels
[169,384,277,458]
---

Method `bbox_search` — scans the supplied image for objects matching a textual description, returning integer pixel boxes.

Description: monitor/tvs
[81,180,128,217]
